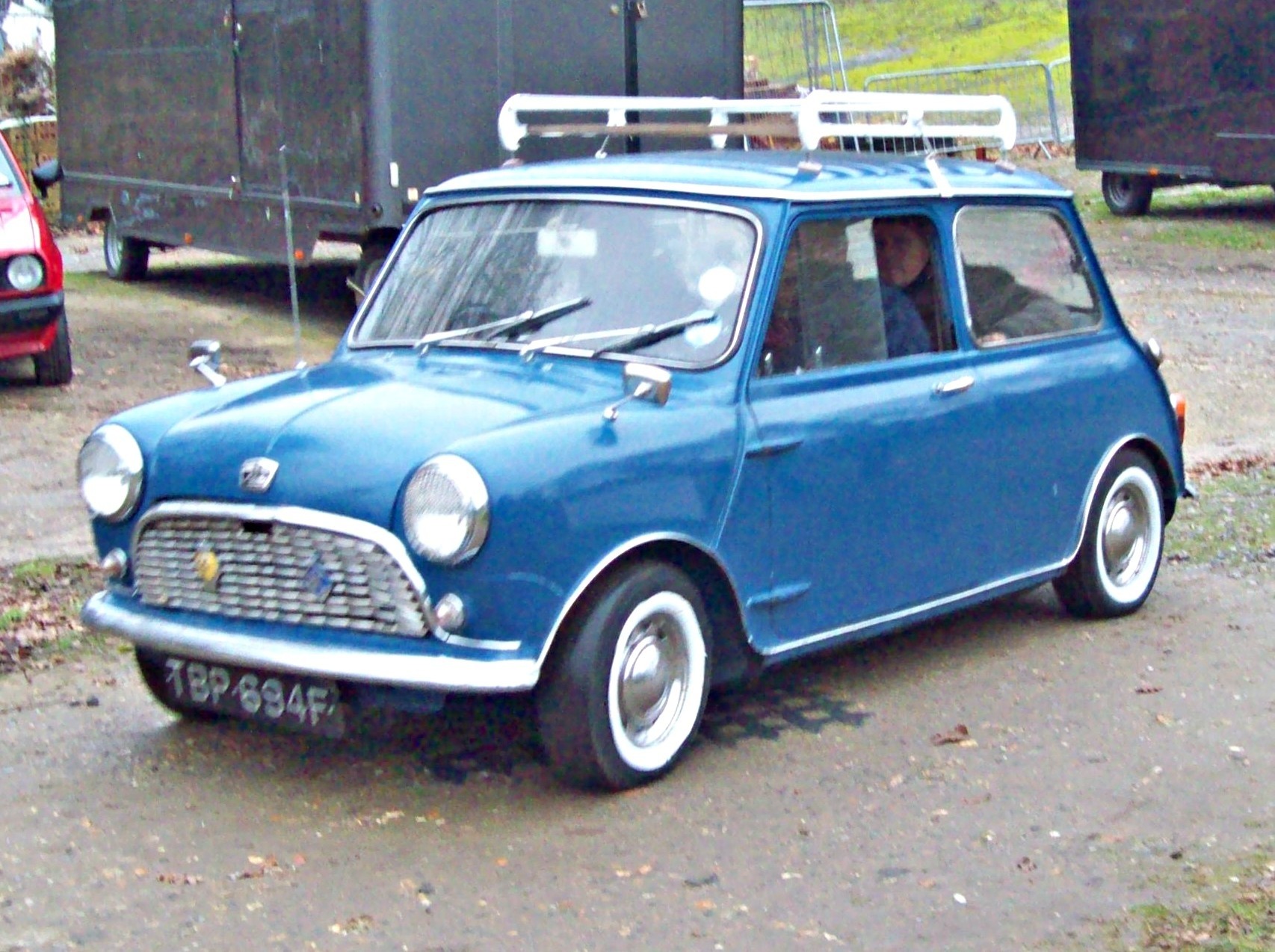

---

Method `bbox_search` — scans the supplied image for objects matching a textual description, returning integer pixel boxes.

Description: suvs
[78,90,1196,796]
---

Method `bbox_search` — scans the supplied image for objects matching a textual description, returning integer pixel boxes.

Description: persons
[737,215,1067,379]
[553,210,714,350]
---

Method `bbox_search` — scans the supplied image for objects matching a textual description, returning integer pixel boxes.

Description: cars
[0,134,72,386]
